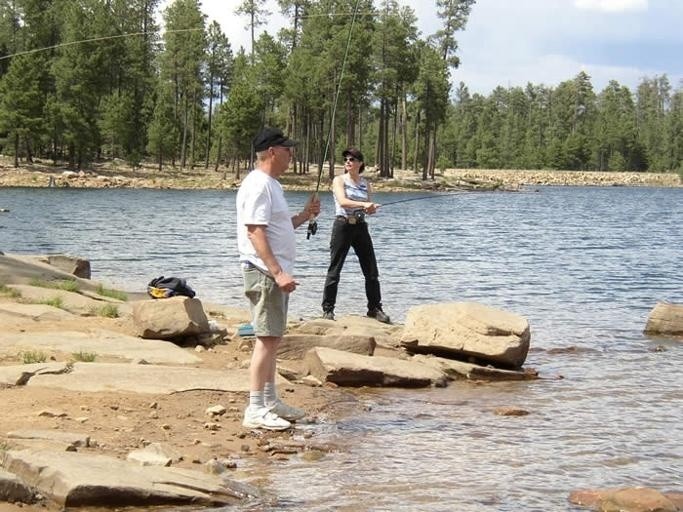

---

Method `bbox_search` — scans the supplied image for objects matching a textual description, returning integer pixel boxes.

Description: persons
[236,128,320,432]
[322,147,391,324]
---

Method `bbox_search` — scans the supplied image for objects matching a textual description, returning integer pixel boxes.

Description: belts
[337,217,364,224]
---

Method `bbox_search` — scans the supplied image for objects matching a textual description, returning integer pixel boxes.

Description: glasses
[343,158,359,161]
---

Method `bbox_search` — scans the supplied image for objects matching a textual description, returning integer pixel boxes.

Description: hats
[342,147,363,161]
[253,128,299,152]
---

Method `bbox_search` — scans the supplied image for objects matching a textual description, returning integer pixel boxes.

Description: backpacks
[145,275,196,299]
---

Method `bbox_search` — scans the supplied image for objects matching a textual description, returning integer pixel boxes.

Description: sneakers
[367,303,389,323]
[243,398,306,431]
[322,305,335,319]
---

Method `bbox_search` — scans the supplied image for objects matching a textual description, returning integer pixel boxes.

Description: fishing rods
[306,1,358,239]
[353,192,480,220]
